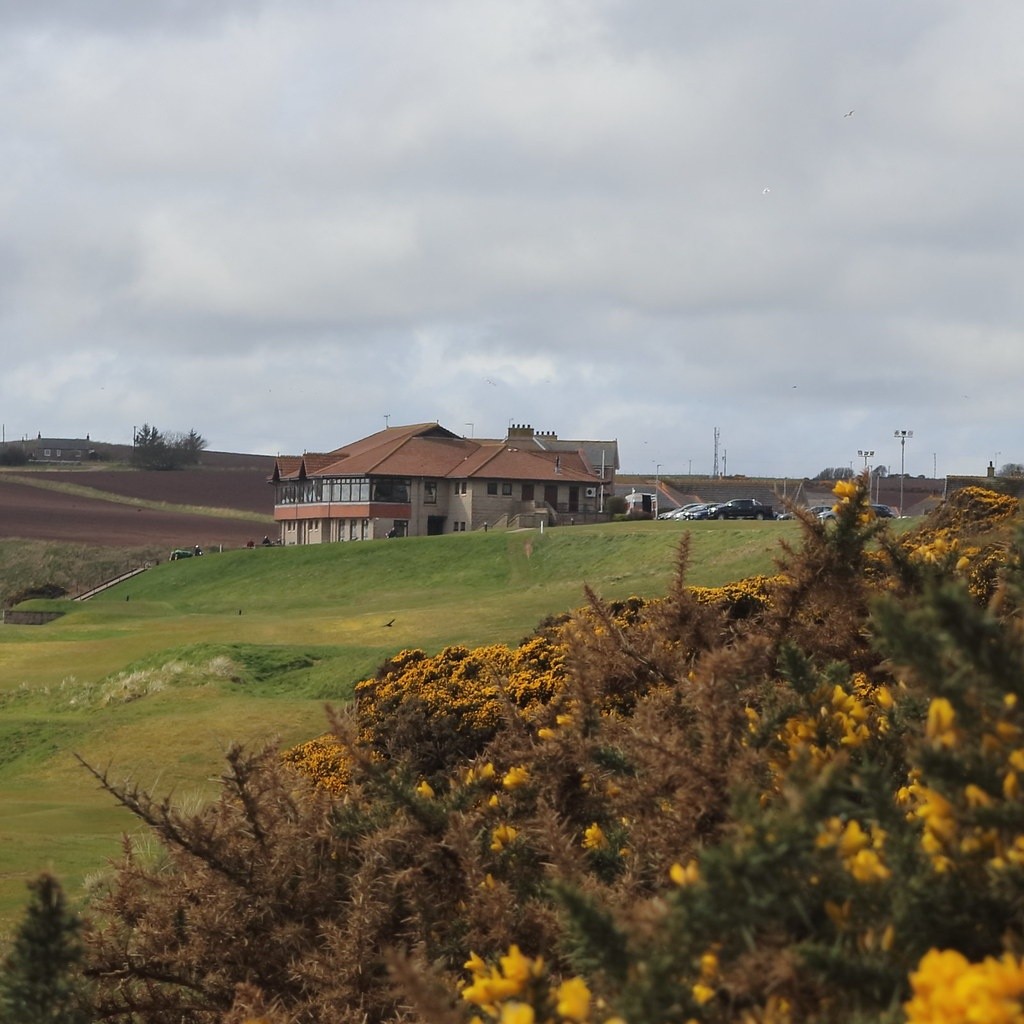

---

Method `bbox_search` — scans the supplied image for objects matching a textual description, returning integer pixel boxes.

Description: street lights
[858,451,874,466]
[894,430,913,518]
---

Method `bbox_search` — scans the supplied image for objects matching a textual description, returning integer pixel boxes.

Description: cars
[777,506,832,520]
[817,508,838,519]
[658,499,773,520]
[872,505,896,519]
